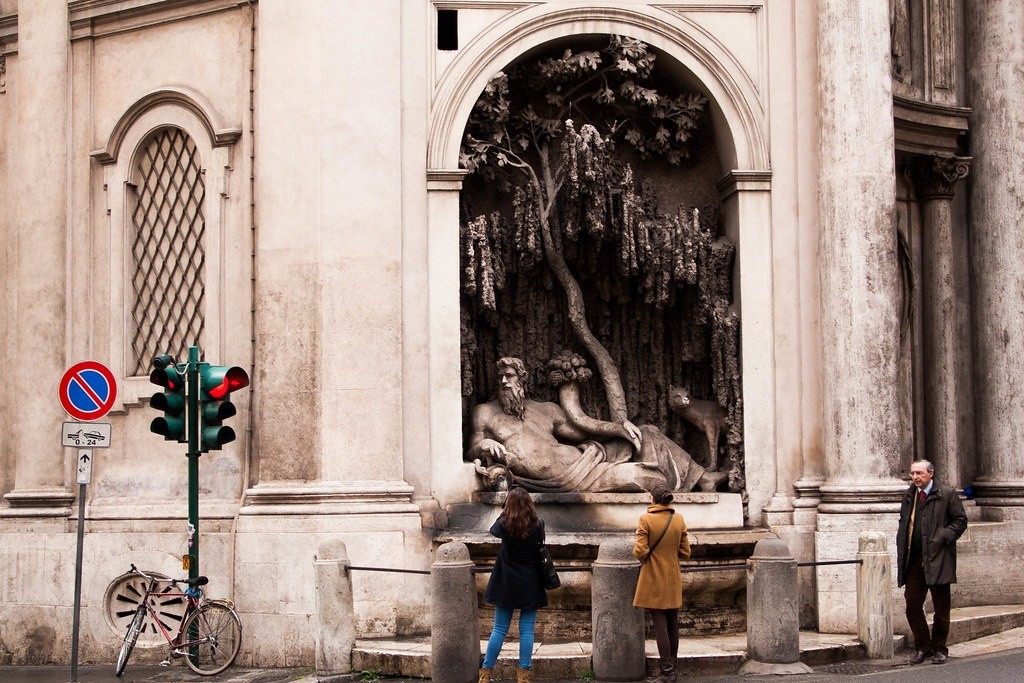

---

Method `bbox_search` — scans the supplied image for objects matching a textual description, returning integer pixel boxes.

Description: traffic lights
[148,357,188,443]
[195,362,250,452]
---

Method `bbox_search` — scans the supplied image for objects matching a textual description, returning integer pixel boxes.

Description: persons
[896,460,969,665]
[632,483,692,683]
[478,488,562,683]
[471,357,729,492]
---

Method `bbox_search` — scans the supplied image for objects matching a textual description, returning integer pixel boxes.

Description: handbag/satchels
[538,517,561,589]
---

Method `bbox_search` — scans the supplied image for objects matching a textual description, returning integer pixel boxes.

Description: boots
[517,667,533,683]
[478,669,492,683]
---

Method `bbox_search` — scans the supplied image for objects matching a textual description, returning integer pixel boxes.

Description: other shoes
[647,676,677,683]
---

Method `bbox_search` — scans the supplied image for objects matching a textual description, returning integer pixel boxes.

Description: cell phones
[512,485,519,490]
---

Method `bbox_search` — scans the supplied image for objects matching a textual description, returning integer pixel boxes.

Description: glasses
[909,472,929,476]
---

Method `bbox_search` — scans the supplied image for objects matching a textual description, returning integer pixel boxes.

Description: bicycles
[115,563,243,674]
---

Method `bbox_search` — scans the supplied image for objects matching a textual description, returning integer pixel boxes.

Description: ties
[920,490,926,506]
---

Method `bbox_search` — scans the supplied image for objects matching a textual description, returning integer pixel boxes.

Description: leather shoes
[910,649,932,664]
[932,651,947,664]
